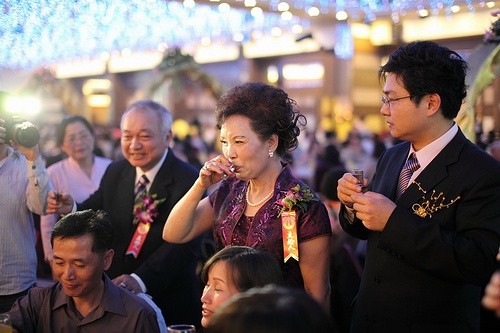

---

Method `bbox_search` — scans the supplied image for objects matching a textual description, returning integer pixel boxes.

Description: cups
[352,169,364,187]
[54,191,63,206]
[217,164,235,180]
[166,324,196,333]
[0,313,12,333]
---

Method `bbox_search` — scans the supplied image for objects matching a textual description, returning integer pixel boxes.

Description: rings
[120,282,127,288]
[207,164,213,170]
[130,290,136,294]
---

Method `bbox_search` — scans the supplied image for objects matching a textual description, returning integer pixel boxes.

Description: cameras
[0,116,40,147]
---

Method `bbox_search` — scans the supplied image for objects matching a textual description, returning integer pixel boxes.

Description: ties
[396,156,421,203]
[133,175,150,209]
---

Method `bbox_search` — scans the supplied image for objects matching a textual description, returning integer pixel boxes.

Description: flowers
[274,184,312,215]
[127,192,165,225]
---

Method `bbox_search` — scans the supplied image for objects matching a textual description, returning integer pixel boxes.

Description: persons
[43,100,215,332]
[37,117,500,201]
[162,82,333,319]
[316,168,362,255]
[40,114,115,282]
[199,245,288,328]
[1,209,162,333]
[1,89,56,315]
[337,41,500,332]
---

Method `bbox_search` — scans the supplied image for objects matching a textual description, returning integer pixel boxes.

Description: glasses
[382,95,413,108]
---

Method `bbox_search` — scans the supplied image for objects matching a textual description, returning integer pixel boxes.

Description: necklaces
[245,184,274,207]
